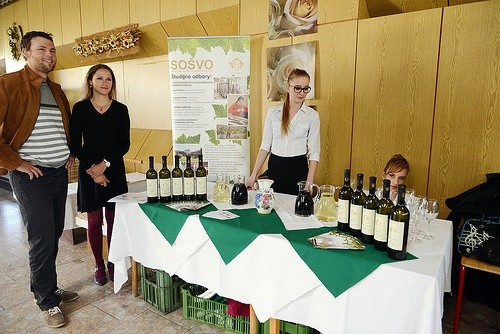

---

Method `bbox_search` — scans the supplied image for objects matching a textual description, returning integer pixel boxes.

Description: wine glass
[405,188,439,243]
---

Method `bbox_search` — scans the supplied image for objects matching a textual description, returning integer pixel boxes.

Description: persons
[355,153,414,209]
[228,95,248,118]
[0,31,79,328]
[68,64,130,285]
[245,68,321,197]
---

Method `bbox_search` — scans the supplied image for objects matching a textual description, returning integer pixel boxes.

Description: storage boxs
[139,265,313,334]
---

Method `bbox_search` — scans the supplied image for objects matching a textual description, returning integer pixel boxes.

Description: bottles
[361,176,380,244]
[373,178,394,251]
[338,169,354,232]
[172,155,183,201]
[254,179,274,214]
[159,156,172,203]
[146,156,158,203]
[183,155,195,201]
[387,183,410,260]
[349,173,368,235]
[195,154,207,201]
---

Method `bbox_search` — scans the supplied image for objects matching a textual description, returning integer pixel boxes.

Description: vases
[255,179,275,214]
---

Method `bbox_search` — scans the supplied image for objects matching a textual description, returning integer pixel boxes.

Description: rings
[91,174,92,176]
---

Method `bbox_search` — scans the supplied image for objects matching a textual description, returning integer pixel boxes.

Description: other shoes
[94,265,107,286]
[41,306,67,328]
[34,287,79,303]
[107,261,114,282]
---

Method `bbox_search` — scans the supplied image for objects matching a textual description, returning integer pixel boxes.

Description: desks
[108,192,452,334]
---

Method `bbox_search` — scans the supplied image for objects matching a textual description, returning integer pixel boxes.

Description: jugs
[315,184,342,224]
[230,174,248,205]
[294,181,319,217]
[213,172,230,203]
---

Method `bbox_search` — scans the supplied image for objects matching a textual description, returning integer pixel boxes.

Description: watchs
[103,158,110,167]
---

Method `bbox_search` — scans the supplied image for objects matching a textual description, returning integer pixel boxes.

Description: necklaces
[91,98,111,111]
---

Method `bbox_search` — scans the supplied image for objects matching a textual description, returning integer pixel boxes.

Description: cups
[349,178,398,205]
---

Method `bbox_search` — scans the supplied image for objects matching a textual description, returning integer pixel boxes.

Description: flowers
[281,0,318,33]
[7,22,20,62]
[72,30,143,58]
[274,54,305,93]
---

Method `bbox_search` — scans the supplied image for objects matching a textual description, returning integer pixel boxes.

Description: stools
[452,255,500,334]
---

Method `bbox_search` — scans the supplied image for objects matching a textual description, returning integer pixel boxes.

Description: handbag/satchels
[457,194,500,265]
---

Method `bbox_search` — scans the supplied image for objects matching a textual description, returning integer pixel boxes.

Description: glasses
[289,85,313,92]
